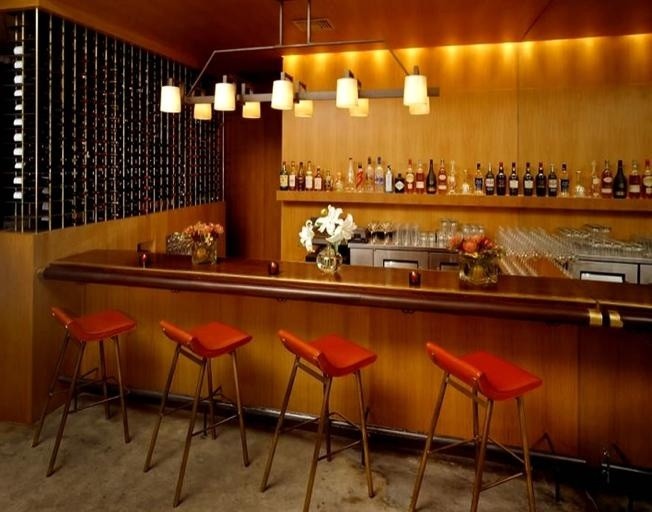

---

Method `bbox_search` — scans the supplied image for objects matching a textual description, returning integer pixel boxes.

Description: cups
[495,221,645,277]
[270,261,279,274]
[391,218,485,252]
[408,271,421,287]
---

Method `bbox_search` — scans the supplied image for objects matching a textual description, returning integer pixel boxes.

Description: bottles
[280,156,651,199]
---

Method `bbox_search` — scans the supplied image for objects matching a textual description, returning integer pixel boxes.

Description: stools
[261,331,377,512]
[31,305,136,476]
[142,319,252,507]
[408,343,542,512]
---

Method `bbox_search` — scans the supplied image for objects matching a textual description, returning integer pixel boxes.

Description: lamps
[159,0,440,119]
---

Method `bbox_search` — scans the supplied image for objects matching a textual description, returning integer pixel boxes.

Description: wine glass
[365,216,391,247]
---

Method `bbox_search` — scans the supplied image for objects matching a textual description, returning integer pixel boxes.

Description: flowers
[183,221,223,246]
[299,205,356,252]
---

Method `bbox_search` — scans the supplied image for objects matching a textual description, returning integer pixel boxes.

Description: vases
[316,243,343,272]
[191,237,216,264]
